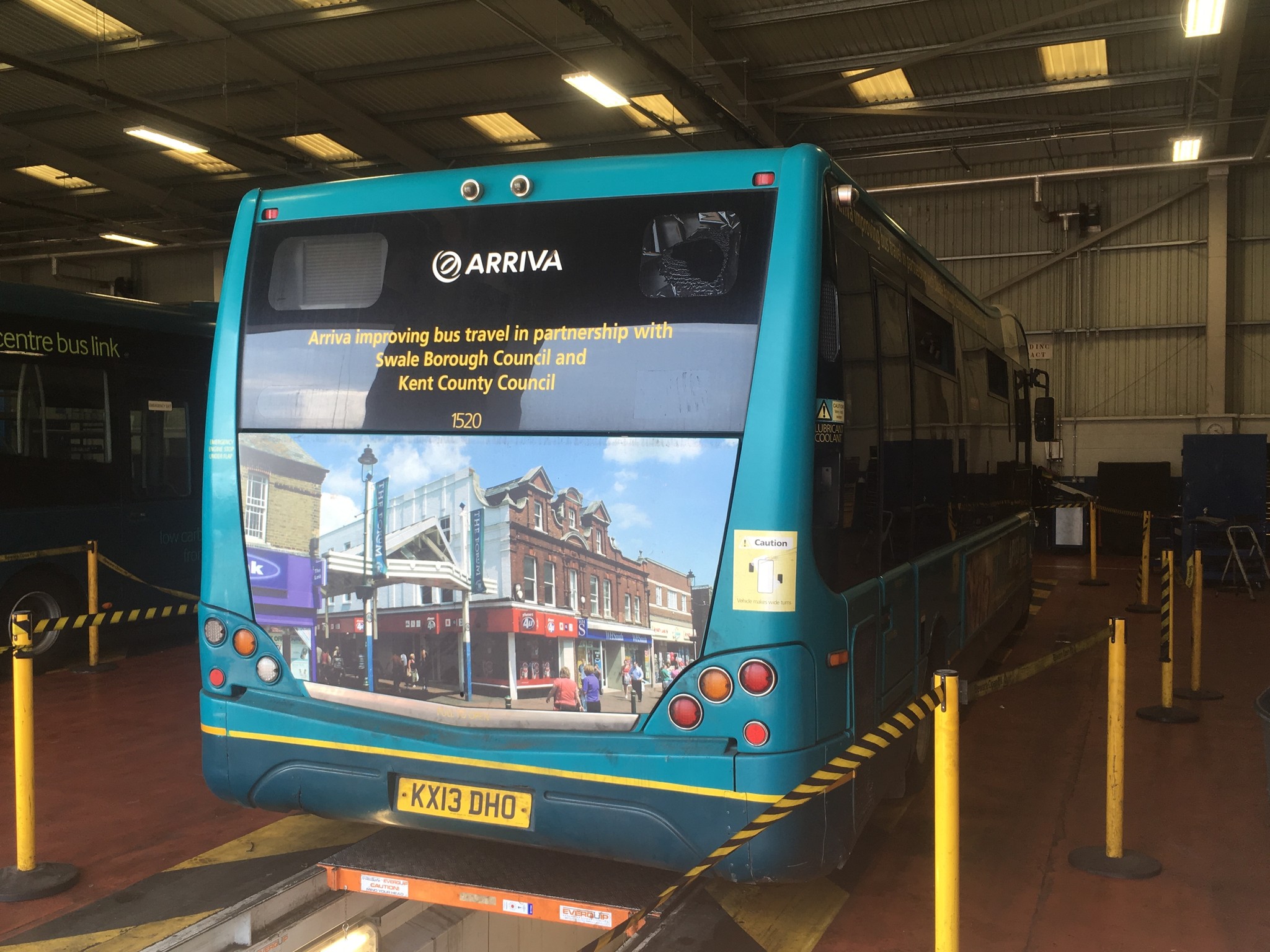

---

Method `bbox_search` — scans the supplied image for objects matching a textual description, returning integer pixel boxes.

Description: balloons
[667,652,682,662]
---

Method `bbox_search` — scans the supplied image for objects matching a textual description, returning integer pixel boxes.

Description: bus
[197,144,1059,886]
[0,278,221,679]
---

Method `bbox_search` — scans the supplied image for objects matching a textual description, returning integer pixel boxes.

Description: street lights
[686,568,697,637]
[356,443,379,693]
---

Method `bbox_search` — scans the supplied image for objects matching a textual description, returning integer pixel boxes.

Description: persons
[629,661,643,702]
[621,659,631,698]
[315,646,430,694]
[659,659,695,692]
[579,658,603,712]
[546,666,585,712]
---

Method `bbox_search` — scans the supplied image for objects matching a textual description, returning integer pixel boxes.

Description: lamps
[123,125,210,155]
[1171,135,1200,162]
[1184,0,1225,37]
[98,232,159,247]
[560,70,632,108]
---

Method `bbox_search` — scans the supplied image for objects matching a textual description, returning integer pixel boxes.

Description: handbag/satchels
[405,668,412,678]
[327,652,332,665]
[554,699,561,708]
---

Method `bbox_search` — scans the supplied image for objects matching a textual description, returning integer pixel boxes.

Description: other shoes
[638,700,641,702]
